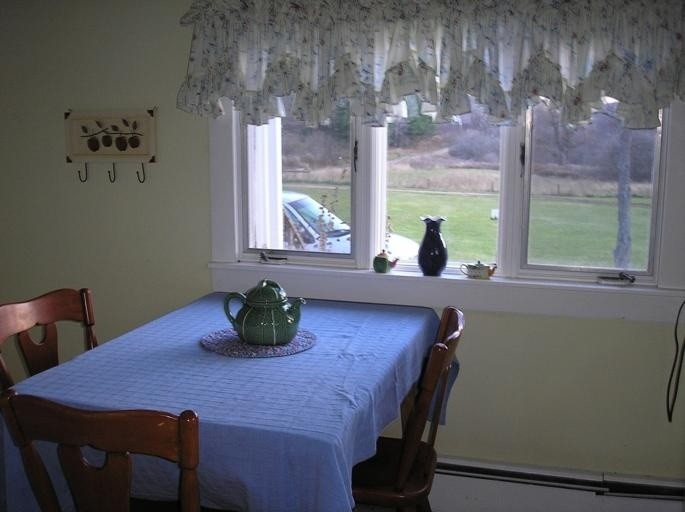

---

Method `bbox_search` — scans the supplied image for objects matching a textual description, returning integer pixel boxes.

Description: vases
[417,217,447,277]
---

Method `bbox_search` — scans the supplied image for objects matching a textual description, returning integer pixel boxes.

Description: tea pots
[459,260,497,280]
[372,249,399,273]
[222,279,306,346]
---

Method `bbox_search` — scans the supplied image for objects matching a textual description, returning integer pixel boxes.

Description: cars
[282,192,421,263]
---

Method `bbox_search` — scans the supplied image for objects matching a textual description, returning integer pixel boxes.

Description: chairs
[0,389,199,512]
[0,289,100,387]
[347,307,461,512]
